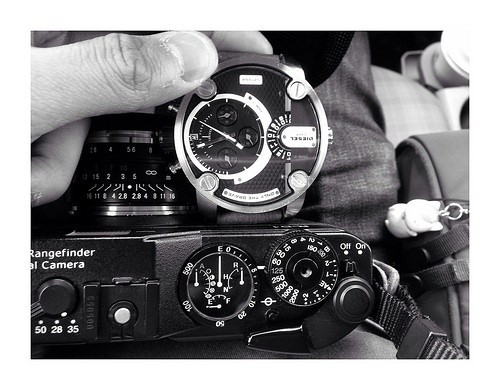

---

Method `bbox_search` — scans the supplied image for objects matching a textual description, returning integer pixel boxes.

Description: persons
[31,31,412,362]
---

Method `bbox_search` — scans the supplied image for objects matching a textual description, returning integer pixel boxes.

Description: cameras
[30,110,375,354]
